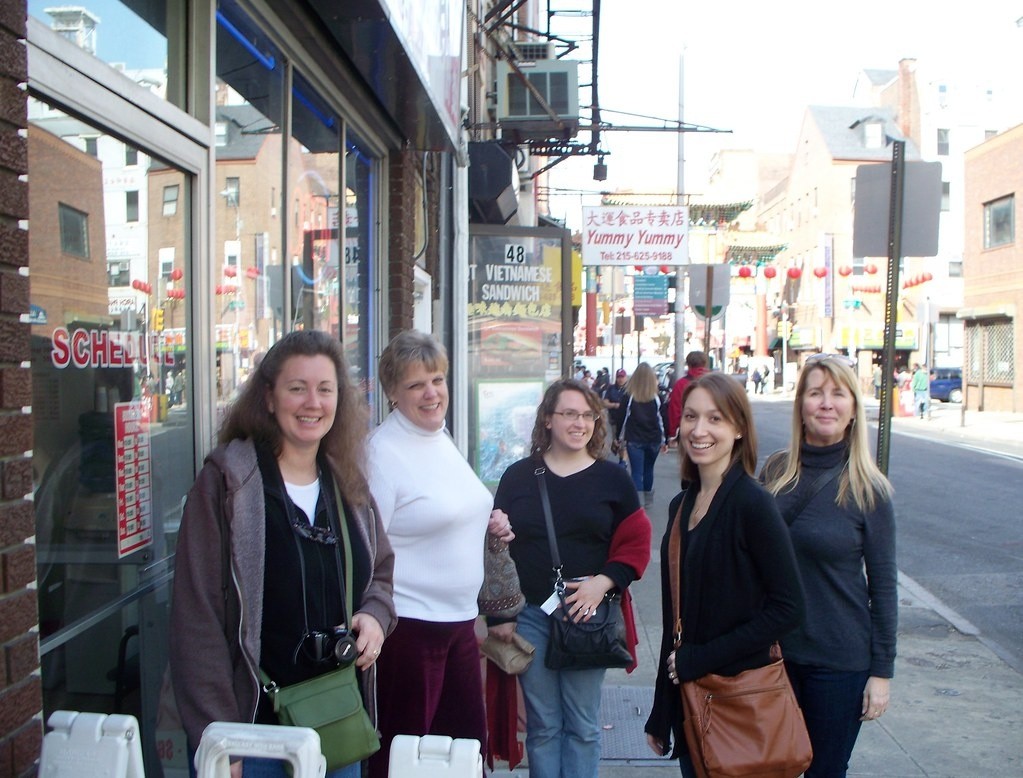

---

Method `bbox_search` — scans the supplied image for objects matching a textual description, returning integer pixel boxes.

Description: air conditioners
[495,60,579,137]
[514,41,557,60]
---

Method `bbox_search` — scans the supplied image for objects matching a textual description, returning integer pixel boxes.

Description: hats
[616,368,627,378]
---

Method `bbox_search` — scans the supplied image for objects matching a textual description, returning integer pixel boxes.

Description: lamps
[528,148,611,181]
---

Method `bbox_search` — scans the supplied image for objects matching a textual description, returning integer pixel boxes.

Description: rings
[373,650,380,656]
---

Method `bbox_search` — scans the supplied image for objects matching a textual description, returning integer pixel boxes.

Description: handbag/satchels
[675,657,813,778]
[543,575,634,671]
[477,528,525,619]
[264,660,382,776]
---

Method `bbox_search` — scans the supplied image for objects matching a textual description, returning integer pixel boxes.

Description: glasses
[806,352,858,368]
[550,407,600,420]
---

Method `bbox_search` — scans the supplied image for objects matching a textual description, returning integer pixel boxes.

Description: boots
[637,489,655,513]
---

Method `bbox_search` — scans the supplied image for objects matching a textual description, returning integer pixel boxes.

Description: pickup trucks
[652,362,749,395]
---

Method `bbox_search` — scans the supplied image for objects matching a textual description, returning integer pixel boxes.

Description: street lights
[219,189,241,389]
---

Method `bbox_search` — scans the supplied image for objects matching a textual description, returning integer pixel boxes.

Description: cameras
[306,628,363,666]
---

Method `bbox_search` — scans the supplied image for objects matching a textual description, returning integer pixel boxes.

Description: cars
[929,368,963,403]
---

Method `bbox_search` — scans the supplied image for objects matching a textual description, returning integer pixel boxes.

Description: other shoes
[619,457,628,468]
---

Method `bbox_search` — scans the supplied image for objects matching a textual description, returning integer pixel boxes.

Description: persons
[490,379,652,778]
[644,373,806,778]
[757,353,898,778]
[172,330,398,778]
[351,329,515,778]
[614,362,668,510]
[874,363,930,417]
[574,366,609,394]
[759,364,770,394]
[604,369,630,440]
[668,351,715,448]
[750,368,762,394]
